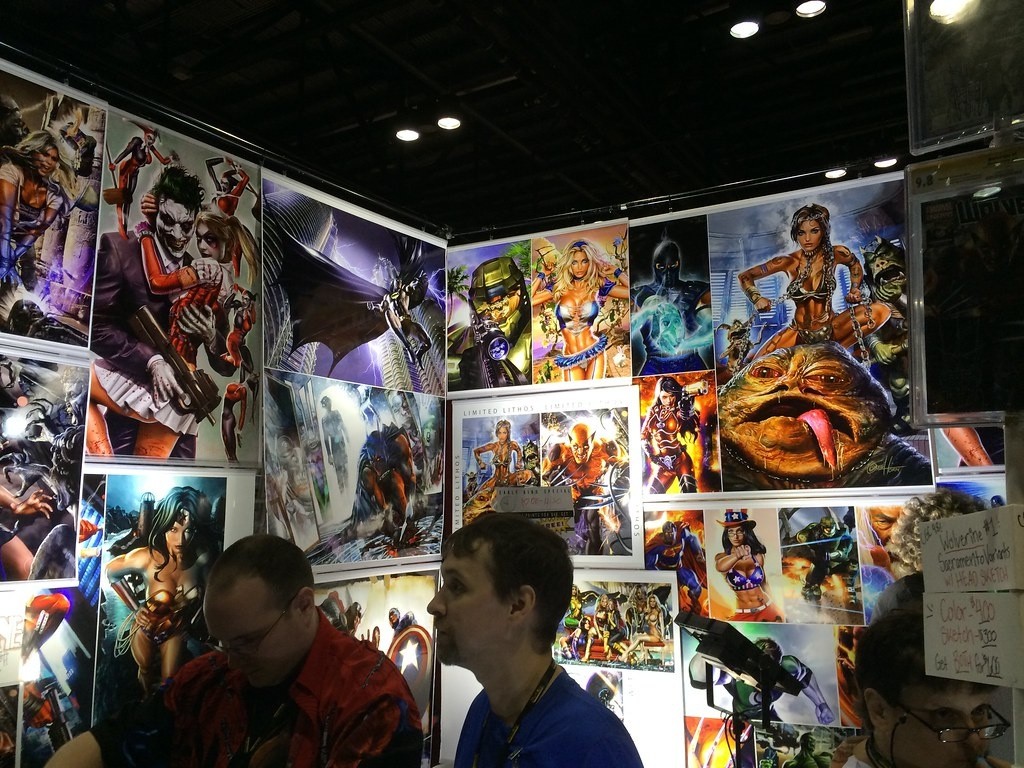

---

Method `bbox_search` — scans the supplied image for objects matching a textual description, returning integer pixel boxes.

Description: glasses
[202,587,301,655]
[727,529,746,537]
[894,699,1011,743]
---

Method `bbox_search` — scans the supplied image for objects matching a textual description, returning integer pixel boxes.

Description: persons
[847,611,1016,768]
[828,572,1015,768]
[41,534,423,768]
[427,512,647,768]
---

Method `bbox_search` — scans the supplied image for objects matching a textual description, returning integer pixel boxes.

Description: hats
[716,509,757,530]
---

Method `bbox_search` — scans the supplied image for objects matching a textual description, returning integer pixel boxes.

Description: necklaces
[865,732,892,768]
